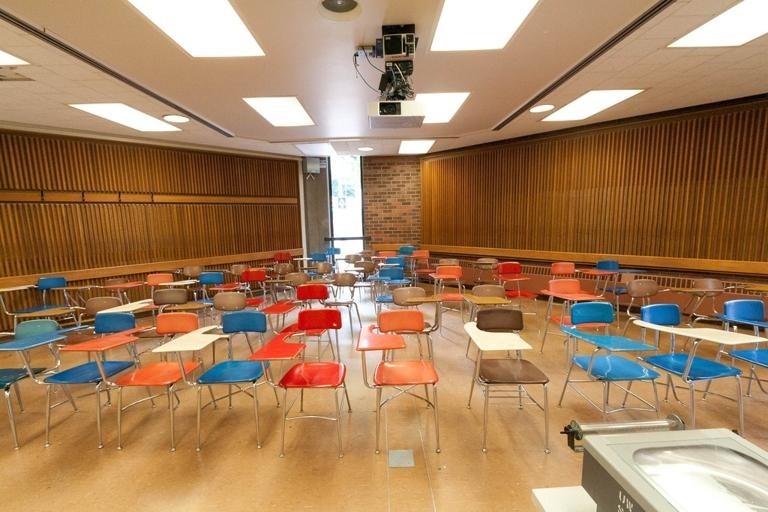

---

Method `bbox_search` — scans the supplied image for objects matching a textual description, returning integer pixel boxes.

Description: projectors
[366,100,426,129]
